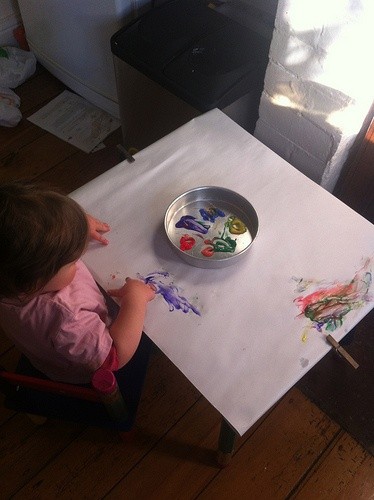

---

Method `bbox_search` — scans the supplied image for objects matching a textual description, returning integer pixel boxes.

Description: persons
[0,184,159,405]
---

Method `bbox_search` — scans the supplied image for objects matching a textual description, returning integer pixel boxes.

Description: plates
[163,184,259,270]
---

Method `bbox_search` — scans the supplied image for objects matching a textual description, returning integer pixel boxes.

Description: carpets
[295,216,373,458]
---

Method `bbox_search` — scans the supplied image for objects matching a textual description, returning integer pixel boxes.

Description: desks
[64,108,374,470]
